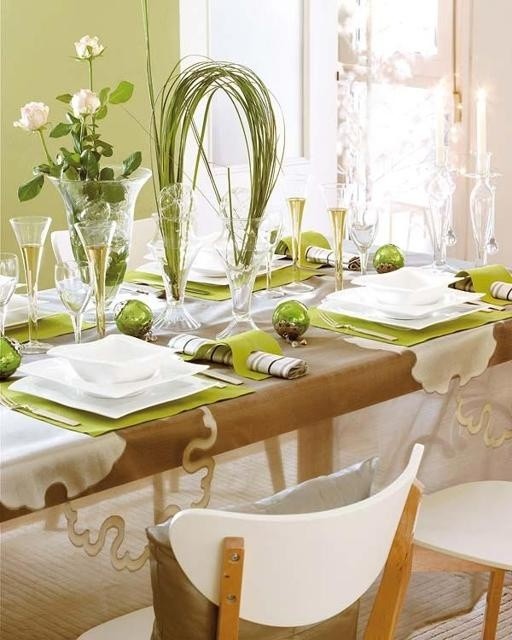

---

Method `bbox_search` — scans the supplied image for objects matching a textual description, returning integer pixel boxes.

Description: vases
[37,167,152,324]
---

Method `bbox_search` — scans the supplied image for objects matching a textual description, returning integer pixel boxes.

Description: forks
[316,310,398,341]
[0,391,80,427]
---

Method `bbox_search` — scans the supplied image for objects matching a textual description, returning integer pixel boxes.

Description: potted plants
[121,0,286,343]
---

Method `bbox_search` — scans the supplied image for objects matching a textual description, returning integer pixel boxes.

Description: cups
[10,216,53,349]
[182,167,281,342]
[279,198,314,294]
[74,219,117,338]
[17,166,154,326]
[320,182,355,291]
[149,165,201,333]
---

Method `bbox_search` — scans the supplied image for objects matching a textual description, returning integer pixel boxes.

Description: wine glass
[345,202,378,273]
[53,260,97,343]
[254,227,284,299]
[1,253,20,305]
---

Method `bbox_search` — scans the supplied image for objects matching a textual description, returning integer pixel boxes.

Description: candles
[434,90,445,166]
[476,89,489,174]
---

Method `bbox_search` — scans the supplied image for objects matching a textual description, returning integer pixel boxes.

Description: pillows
[146,456,381,640]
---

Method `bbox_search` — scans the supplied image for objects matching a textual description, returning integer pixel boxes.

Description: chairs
[412,480,512,640]
[74,443,426,640]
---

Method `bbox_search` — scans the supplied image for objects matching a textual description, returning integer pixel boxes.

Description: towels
[284,245,361,271]
[449,278,512,302]
[167,333,310,379]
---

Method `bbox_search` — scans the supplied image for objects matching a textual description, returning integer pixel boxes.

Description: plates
[316,266,491,330]
[8,333,218,420]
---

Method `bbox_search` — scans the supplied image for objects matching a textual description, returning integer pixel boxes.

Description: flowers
[12,35,144,286]
